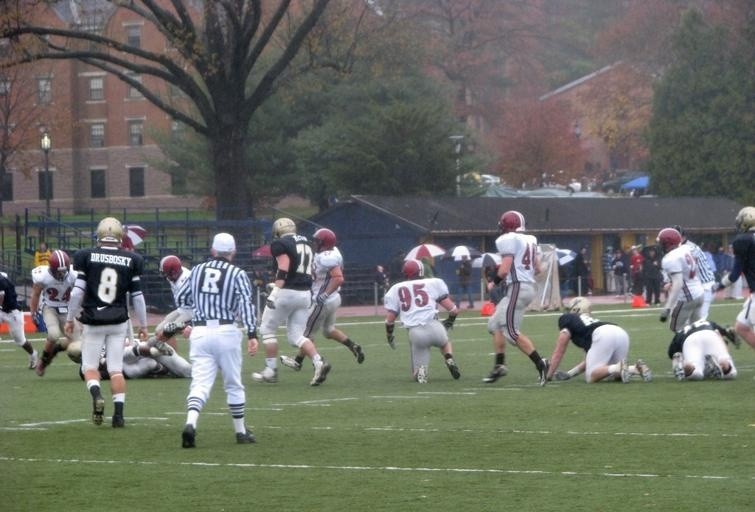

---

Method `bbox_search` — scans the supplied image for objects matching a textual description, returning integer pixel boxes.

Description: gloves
[555,370,569,379]
[441,318,454,331]
[711,280,726,294]
[387,334,396,351]
[318,292,328,306]
[659,309,670,322]
[266,286,280,310]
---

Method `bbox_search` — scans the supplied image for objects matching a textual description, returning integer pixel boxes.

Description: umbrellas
[404,242,447,262]
[120,225,146,248]
[440,244,483,258]
[557,248,576,266]
[470,251,503,269]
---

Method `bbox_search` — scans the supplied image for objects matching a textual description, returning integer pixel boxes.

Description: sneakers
[482,365,508,383]
[538,358,549,386]
[726,327,740,350]
[353,343,365,363]
[280,355,302,371]
[182,424,196,448]
[417,365,428,384]
[310,358,331,386]
[36,359,44,375]
[704,354,724,380]
[672,352,685,382]
[236,430,256,443]
[29,350,39,368]
[252,367,280,383]
[112,416,124,427]
[163,320,186,334]
[619,358,629,383]
[636,359,652,382]
[445,358,461,379]
[93,396,106,425]
[154,341,174,356]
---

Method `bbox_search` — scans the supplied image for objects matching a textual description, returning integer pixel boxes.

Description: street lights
[41,133,52,248]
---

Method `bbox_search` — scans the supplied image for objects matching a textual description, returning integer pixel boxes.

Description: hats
[213,233,236,252]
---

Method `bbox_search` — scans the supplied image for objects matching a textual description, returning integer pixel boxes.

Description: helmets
[313,229,336,252]
[401,260,424,279]
[734,207,755,231]
[50,250,70,280]
[566,297,591,314]
[272,217,296,238]
[160,256,182,277]
[656,228,681,249]
[498,211,525,232]
[97,217,123,244]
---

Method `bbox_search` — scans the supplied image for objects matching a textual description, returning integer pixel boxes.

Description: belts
[194,320,233,326]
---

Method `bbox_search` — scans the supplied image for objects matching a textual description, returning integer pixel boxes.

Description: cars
[619,174,650,197]
[602,176,632,194]
[541,172,582,193]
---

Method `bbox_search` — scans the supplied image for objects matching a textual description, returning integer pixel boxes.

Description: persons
[724,242,744,301]
[601,246,616,292]
[671,225,715,324]
[611,249,629,294]
[64,217,148,429]
[487,266,504,305]
[655,228,703,334]
[456,255,475,308]
[481,210,549,386]
[252,269,275,313]
[30,250,79,376]
[159,255,192,352]
[375,265,391,304]
[546,296,653,382]
[575,247,593,296]
[712,245,729,289]
[251,218,331,386]
[629,246,646,292]
[384,258,461,383]
[668,319,741,380]
[280,228,365,371]
[174,233,258,448]
[0,271,39,369]
[69,333,190,382]
[34,242,52,267]
[643,249,662,306]
[713,207,754,348]
